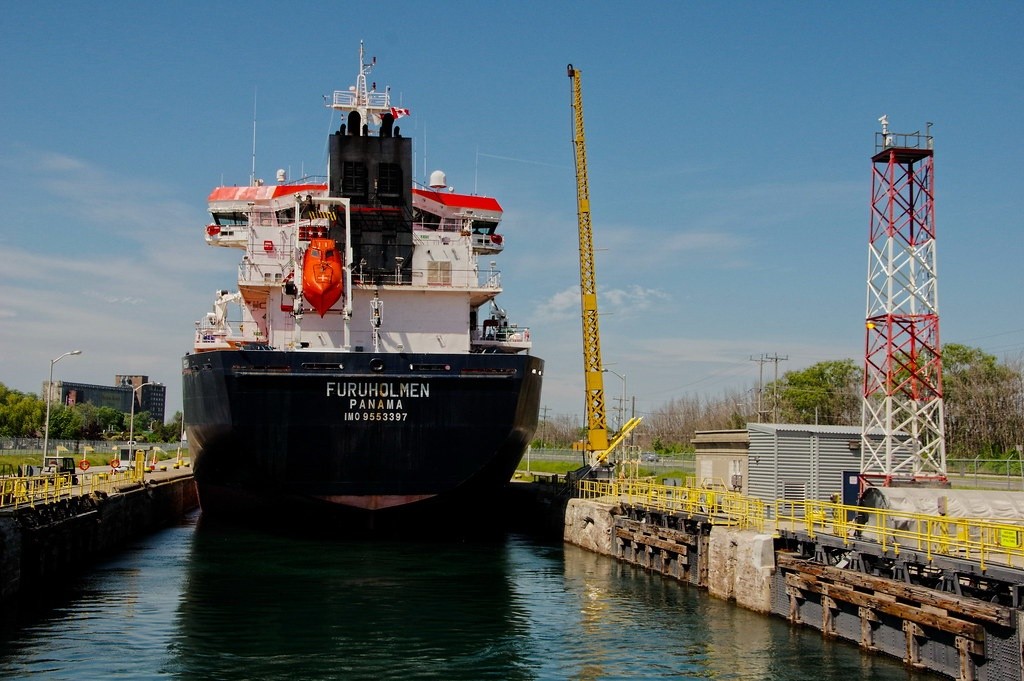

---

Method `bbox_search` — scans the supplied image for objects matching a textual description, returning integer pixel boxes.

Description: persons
[473,227,481,234]
[300,195,315,218]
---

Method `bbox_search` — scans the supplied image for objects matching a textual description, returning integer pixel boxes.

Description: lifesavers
[110,459,120,468]
[78,460,90,470]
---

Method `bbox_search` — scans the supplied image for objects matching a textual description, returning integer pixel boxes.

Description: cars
[36,455,79,484]
[0,461,29,498]
[641,454,660,462]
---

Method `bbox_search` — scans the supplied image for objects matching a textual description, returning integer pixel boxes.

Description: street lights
[40,349,83,484]
[600,367,627,475]
[120,380,152,470]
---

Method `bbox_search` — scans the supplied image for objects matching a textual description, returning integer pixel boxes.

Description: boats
[179,38,546,527]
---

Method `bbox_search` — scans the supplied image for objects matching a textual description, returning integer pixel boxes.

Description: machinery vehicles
[564,61,616,489]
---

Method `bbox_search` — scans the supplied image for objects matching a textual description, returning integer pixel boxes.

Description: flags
[392,107,409,119]
